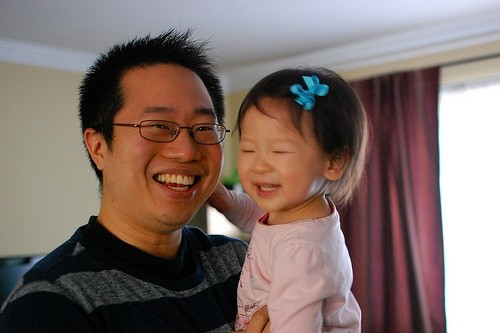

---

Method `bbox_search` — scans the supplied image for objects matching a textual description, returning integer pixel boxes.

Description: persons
[205,67,374,333]
[0,27,270,333]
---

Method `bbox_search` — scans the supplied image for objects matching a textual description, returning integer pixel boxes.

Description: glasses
[91,120,230,144]
